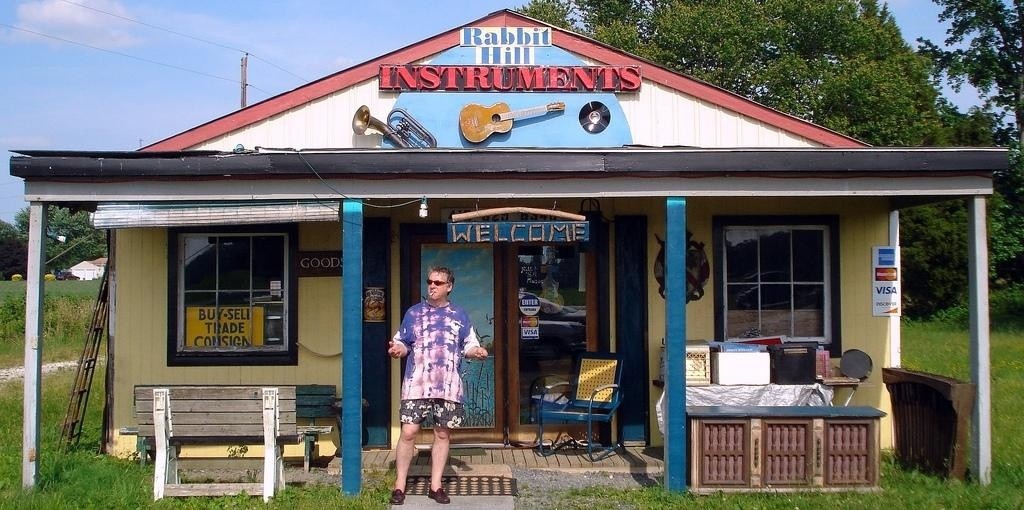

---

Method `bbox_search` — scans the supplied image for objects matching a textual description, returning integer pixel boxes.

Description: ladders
[54,256,108,455]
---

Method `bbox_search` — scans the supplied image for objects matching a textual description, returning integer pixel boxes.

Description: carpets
[405,475,519,496]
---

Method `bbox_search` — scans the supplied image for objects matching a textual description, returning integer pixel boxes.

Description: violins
[539,247,564,314]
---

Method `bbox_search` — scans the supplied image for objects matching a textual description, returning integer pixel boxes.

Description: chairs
[527,373,573,425]
[539,351,628,462]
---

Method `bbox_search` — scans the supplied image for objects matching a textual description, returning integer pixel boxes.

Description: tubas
[352,105,438,149]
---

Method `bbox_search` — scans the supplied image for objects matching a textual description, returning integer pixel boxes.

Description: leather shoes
[429,486,450,504]
[391,486,406,505]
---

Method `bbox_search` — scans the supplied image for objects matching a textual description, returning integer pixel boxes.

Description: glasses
[427,278,448,286]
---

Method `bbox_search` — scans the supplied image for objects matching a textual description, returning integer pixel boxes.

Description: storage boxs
[774,348,816,384]
[710,351,770,385]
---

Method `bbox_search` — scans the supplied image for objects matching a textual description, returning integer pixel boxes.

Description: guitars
[460,101,565,143]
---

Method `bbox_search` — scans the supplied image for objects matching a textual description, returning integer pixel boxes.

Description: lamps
[418,195,431,218]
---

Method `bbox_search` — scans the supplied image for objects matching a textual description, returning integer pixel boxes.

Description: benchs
[117,383,336,471]
[134,386,298,501]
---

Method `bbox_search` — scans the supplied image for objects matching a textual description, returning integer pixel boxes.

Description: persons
[386,264,489,505]
